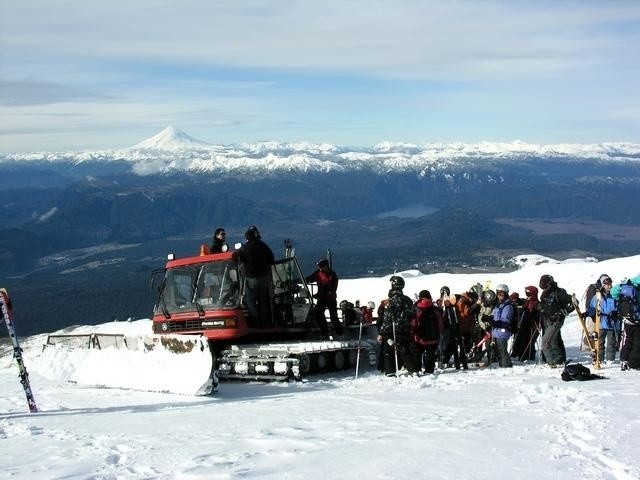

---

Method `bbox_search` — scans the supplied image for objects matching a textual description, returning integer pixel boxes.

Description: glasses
[221,232,225,236]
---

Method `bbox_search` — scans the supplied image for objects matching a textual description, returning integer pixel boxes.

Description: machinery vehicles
[149,231,380,384]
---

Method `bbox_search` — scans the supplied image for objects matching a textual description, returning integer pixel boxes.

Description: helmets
[387,274,640,301]
[339,298,376,312]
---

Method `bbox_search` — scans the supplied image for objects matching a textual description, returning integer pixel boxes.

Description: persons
[210,227,230,253]
[232,226,275,327]
[243,225,263,245]
[285,258,341,340]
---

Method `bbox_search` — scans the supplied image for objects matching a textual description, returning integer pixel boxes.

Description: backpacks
[618,284,638,317]
[561,363,603,381]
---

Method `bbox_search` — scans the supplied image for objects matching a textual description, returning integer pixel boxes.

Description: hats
[316,257,328,267]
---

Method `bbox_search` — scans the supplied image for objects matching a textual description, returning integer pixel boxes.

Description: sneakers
[374,355,640,379]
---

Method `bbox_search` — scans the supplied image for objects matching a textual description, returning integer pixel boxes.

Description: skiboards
[570,289,603,371]
[0,288,39,413]
